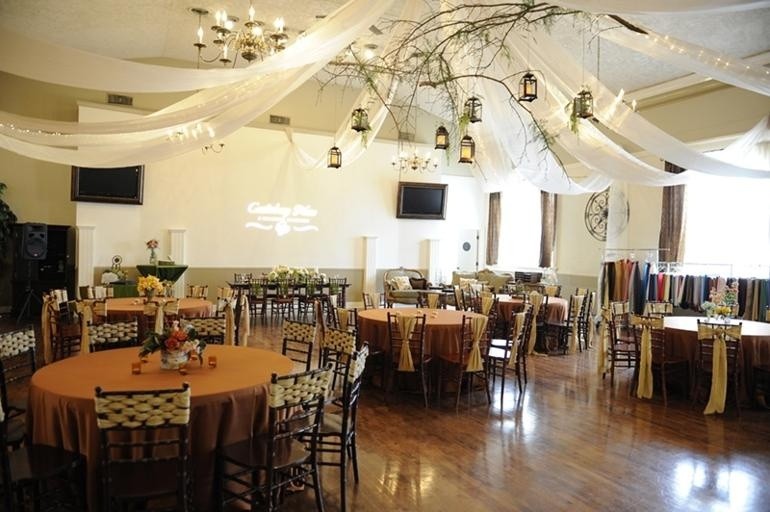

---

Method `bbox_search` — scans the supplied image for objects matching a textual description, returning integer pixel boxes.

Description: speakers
[20,222,48,260]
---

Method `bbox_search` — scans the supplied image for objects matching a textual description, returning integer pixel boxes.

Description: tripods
[15,260,43,325]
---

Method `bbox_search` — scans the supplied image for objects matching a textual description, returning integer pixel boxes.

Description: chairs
[754,365,769,408]
[645,301,673,316]
[227,272,351,326]
[281,320,315,371]
[437,315,491,411]
[88,317,140,352]
[383,267,568,348]
[1,370,87,509]
[363,292,374,310]
[602,300,641,378]
[691,319,741,414]
[1,325,37,413]
[576,288,594,353]
[43,287,83,361]
[94,383,190,511]
[304,328,368,510]
[385,313,429,407]
[544,296,583,353]
[486,306,533,392]
[79,285,114,300]
[630,311,690,405]
[215,364,324,510]
[178,317,226,347]
[191,285,206,299]
[334,308,358,338]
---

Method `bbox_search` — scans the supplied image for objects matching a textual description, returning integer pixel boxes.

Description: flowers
[136,276,163,296]
[699,277,739,317]
[267,265,323,282]
[141,327,206,355]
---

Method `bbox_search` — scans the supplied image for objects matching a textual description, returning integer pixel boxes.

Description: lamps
[191,0,292,69]
[574,21,593,118]
[328,108,341,167]
[459,122,475,163]
[436,126,449,150]
[465,98,482,122]
[166,113,226,158]
[351,109,370,133]
[519,24,537,102]
[392,139,438,174]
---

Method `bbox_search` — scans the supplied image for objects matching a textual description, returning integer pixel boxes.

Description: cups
[131,351,217,375]
